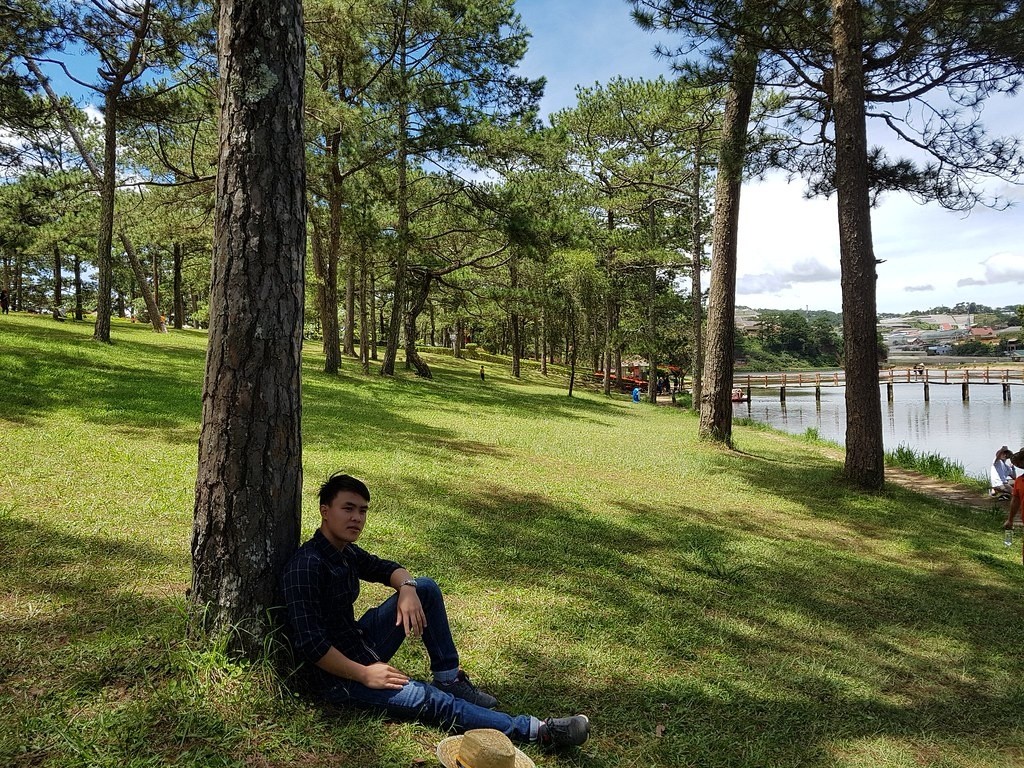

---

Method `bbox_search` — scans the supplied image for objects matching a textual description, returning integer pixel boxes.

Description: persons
[1003,446,1024,563]
[990,446,1016,496]
[0,289,10,315]
[912,362,925,375]
[480,365,485,382]
[272,470,592,754]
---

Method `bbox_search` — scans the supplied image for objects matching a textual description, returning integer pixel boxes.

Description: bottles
[1005,528,1013,545]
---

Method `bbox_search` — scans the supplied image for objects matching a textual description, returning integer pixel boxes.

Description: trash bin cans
[632,387,641,401]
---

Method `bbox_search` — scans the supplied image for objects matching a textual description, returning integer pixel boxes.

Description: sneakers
[537,715,590,747]
[430,669,498,709]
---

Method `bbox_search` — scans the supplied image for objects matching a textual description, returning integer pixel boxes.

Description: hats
[996,446,1013,459]
[435,729,536,768]
[1011,447,1024,470]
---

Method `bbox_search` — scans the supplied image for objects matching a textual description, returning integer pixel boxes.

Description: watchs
[398,579,418,587]
